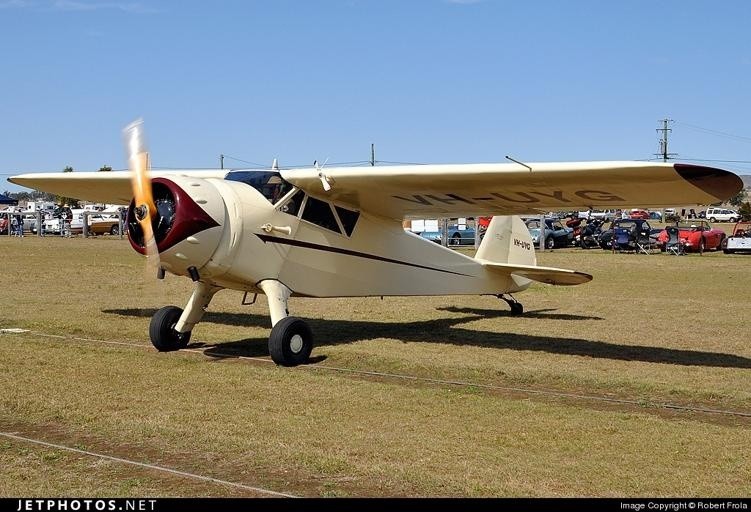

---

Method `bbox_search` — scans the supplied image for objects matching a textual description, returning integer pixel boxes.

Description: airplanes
[6,118,743,368]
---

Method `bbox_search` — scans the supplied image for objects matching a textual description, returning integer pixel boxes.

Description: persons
[686,208,698,227]
[121,206,129,236]
[36,206,46,233]
[673,212,680,223]
[63,203,73,238]
[53,202,64,237]
[15,208,24,237]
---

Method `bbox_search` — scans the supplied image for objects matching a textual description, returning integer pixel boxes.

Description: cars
[0,194,128,236]
